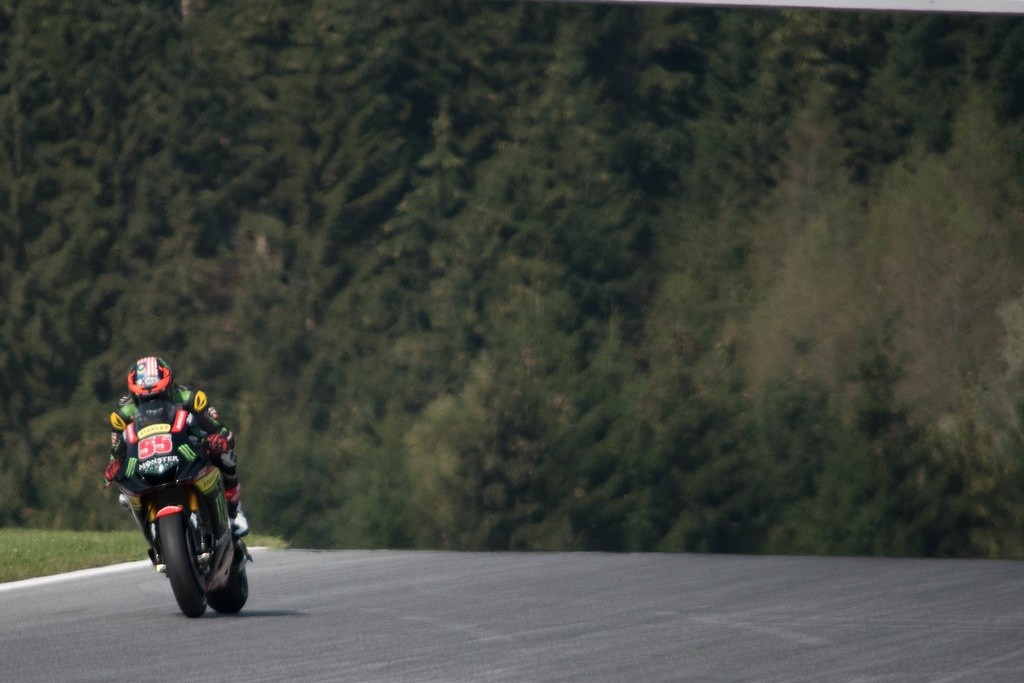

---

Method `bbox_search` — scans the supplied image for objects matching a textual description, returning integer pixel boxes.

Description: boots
[224,484,249,536]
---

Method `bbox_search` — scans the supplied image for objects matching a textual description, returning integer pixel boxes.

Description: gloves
[206,433,228,453]
[104,459,122,482]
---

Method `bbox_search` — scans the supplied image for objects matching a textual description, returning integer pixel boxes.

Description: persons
[104,357,249,574]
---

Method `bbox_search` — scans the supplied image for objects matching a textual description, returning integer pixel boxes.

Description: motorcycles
[109,388,254,618]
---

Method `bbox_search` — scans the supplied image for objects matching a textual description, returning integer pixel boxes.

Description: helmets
[127,356,176,416]
